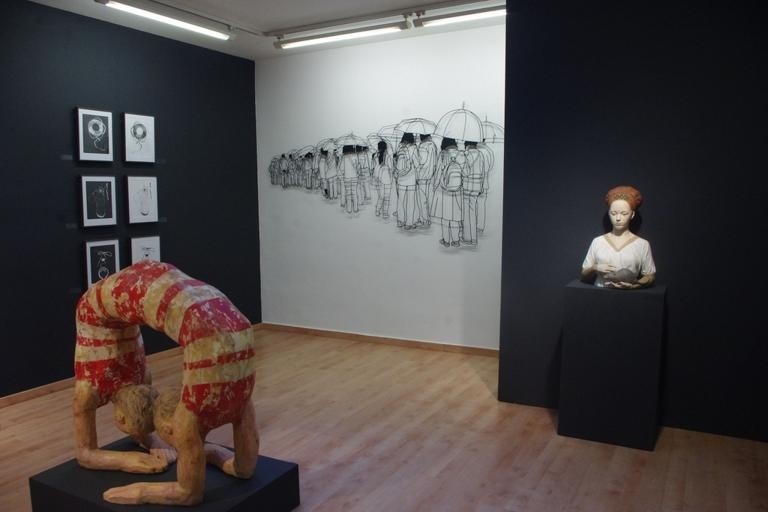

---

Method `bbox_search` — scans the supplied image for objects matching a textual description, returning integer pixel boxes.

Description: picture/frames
[80,175,118,227]
[85,238,121,289]
[75,106,113,163]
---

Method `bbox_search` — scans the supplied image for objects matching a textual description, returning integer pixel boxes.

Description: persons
[579,186,659,290]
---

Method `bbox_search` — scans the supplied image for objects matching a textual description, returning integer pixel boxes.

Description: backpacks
[442,149,463,194]
[378,152,392,185]
[396,143,414,177]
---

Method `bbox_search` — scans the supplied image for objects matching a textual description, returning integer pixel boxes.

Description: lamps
[414,3,507,29]
[273,12,412,51]
[93,0,237,42]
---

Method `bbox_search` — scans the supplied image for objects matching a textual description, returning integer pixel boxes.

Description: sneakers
[340,203,484,247]
